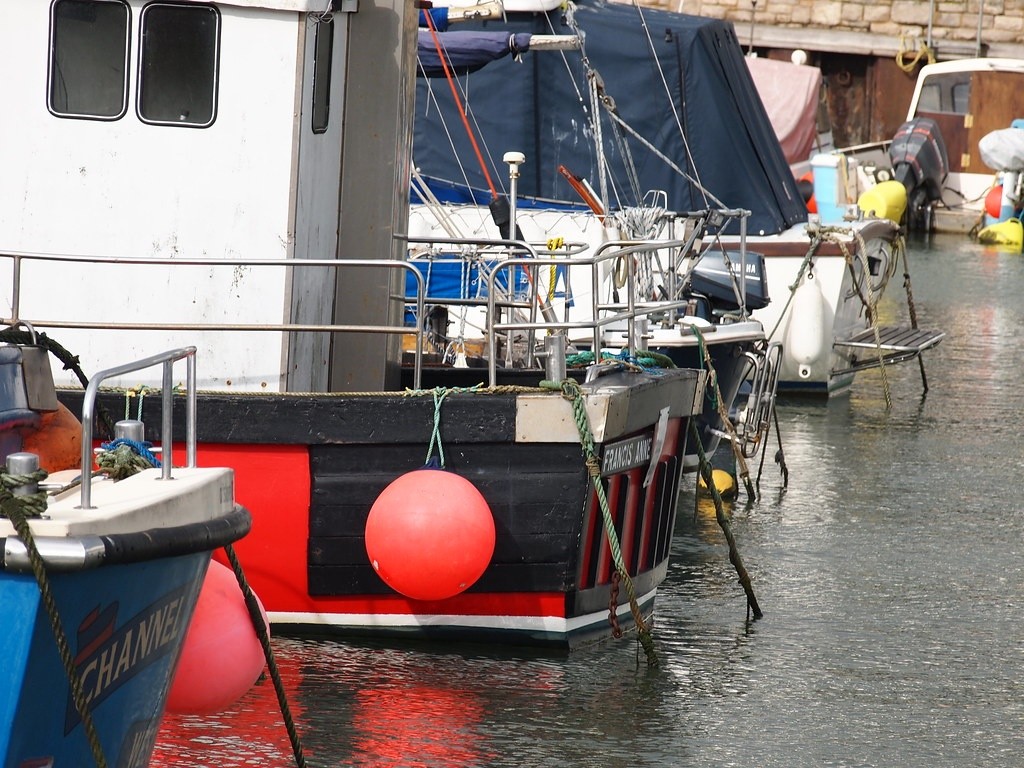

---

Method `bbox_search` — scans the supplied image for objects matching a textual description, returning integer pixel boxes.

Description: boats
[829,58,1024,244]
[0,0,946,651]
[0,320,250,768]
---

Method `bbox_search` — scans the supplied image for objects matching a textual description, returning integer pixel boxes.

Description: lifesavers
[614,246,629,286]
